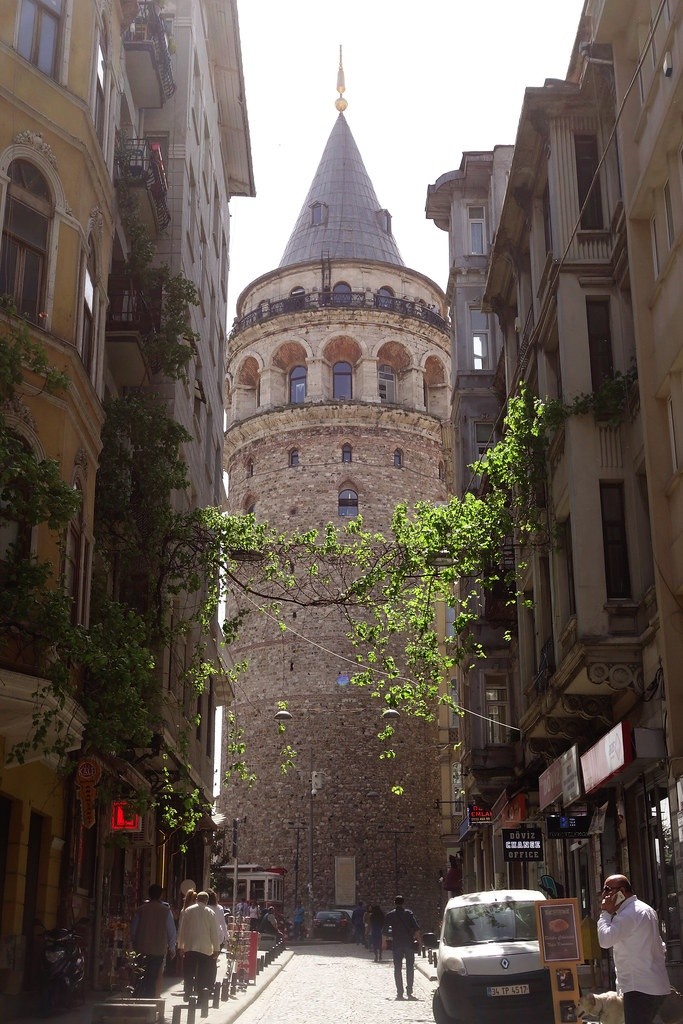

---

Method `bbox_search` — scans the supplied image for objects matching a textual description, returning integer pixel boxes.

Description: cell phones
[609,891,625,906]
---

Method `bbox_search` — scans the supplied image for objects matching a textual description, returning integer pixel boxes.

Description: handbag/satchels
[413,939,419,951]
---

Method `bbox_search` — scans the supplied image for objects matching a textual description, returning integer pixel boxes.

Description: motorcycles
[33,918,89,1015]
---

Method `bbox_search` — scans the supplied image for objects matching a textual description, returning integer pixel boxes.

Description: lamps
[383,708,399,720]
[275,709,293,721]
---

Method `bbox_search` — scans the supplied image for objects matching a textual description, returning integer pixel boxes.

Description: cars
[312,911,353,941]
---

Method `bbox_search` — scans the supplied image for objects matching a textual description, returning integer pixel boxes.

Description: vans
[420,889,555,1024]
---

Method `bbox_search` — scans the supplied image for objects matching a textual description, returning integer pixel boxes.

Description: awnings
[160,793,219,855]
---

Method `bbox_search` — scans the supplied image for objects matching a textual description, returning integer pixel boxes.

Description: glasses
[603,885,619,893]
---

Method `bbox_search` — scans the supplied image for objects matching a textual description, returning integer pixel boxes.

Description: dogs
[572,991,662,1024]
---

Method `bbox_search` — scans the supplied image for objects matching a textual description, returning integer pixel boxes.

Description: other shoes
[397,994,403,999]
[183,991,191,1001]
[408,989,413,995]
[375,957,382,961]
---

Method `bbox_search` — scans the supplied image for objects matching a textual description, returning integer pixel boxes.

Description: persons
[293,901,304,941]
[179,890,199,981]
[205,888,228,990]
[370,903,384,962]
[363,904,373,948]
[129,884,176,999]
[597,875,670,1024]
[177,892,220,1002]
[351,900,364,945]
[235,895,284,946]
[384,895,422,997]
